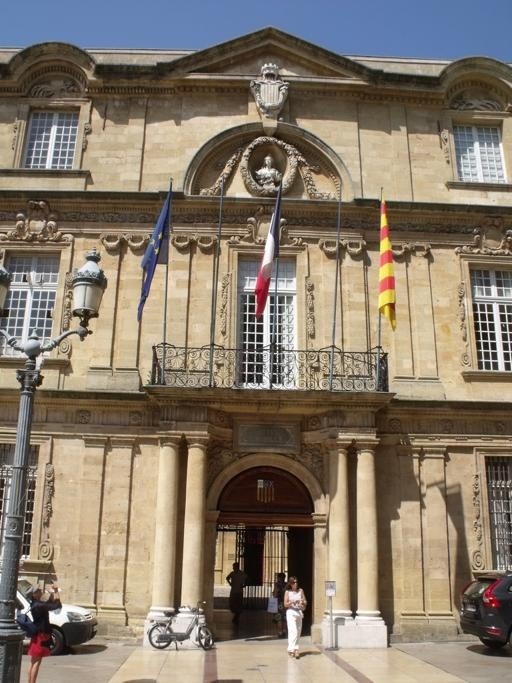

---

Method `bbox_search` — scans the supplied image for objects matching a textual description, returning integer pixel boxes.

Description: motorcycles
[146,600,215,651]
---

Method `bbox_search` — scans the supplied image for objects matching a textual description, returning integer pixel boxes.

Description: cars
[14,579,98,655]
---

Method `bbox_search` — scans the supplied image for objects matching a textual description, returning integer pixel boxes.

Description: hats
[25,584,41,599]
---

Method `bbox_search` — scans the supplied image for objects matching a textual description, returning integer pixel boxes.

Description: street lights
[0,250,109,683]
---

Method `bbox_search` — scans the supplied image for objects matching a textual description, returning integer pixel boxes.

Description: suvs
[460,570,512,649]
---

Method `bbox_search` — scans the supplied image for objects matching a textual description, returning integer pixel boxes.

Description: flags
[253,191,280,320]
[378,190,397,332]
[136,190,171,320]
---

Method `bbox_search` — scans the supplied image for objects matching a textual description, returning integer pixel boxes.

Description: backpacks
[17,613,38,636]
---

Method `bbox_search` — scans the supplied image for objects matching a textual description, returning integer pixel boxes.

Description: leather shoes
[295,650,299,659]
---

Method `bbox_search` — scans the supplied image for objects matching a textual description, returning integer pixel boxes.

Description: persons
[272,571,291,638]
[255,154,281,189]
[283,574,307,659]
[225,561,249,623]
[27,580,62,682]
